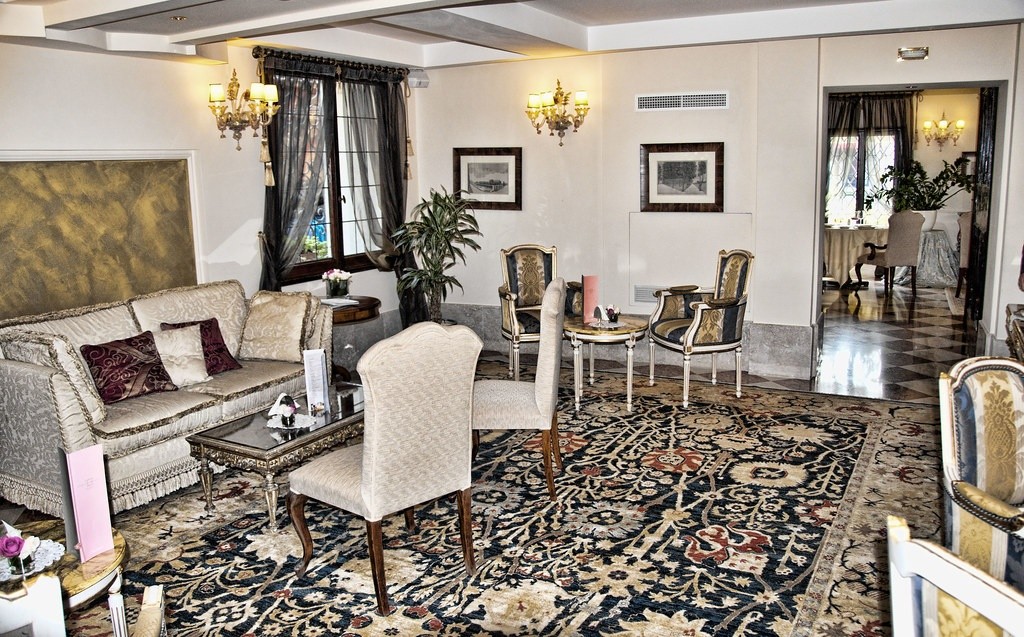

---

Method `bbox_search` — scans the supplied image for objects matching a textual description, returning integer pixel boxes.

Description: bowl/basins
[825,224,833,228]
[859,227,875,229]
[840,227,849,229]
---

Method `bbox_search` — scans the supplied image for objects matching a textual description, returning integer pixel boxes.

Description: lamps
[922,111,964,151]
[525,79,590,146]
[209,69,281,151]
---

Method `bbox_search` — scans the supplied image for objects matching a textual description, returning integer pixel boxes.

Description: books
[319,297,359,307]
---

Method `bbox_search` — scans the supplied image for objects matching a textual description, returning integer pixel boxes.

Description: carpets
[17,360,945,637]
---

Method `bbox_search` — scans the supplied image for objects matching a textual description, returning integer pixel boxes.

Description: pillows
[78,316,244,404]
[2,329,107,424]
[236,291,321,364]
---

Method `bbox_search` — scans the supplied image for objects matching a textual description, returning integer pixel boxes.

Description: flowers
[606,304,621,316]
[321,268,352,281]
[0,520,41,558]
[268,392,301,417]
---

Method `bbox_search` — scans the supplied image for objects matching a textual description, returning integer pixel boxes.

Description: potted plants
[390,184,485,327]
[865,153,981,232]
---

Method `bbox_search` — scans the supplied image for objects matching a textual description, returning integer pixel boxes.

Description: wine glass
[851,211,863,224]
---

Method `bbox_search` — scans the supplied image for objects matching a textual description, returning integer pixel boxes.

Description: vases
[281,414,295,426]
[8,551,35,575]
[609,314,619,322]
[326,279,348,296]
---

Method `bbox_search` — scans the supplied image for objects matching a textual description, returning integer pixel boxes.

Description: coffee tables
[186,381,364,527]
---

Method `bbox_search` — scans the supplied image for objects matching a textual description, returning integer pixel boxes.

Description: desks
[0,520,125,637]
[564,315,648,413]
[823,226,887,292]
[317,296,381,325]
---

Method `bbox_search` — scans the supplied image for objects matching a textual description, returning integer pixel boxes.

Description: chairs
[955,211,971,298]
[287,322,482,616]
[856,210,926,296]
[887,353,1024,637]
[472,278,565,500]
[647,249,756,409]
[497,243,567,381]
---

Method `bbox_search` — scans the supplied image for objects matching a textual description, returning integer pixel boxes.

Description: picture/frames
[453,147,522,210]
[639,142,723,212]
[963,151,977,183]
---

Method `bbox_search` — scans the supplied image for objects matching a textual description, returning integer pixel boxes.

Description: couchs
[0,279,333,523]
